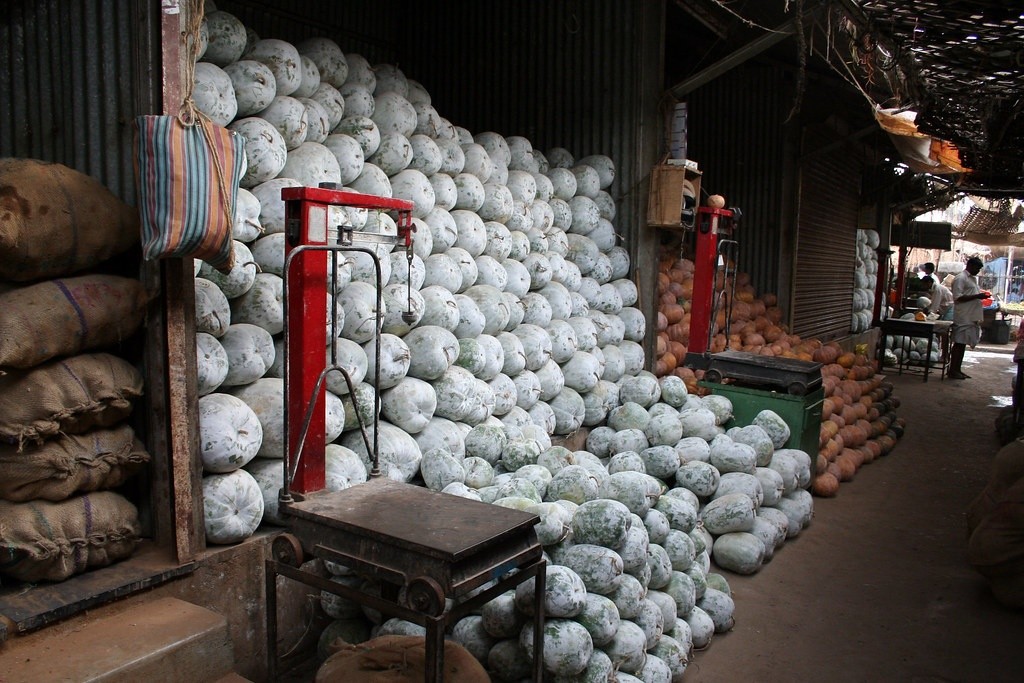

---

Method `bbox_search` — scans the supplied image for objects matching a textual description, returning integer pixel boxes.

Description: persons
[924,263,940,284]
[920,275,954,362]
[917,264,928,278]
[1008,288,1020,303]
[947,257,991,380]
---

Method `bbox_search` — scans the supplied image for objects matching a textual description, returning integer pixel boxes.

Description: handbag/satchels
[128,112,246,276]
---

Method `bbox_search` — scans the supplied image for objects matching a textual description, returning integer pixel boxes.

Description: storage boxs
[666,158,698,169]
[646,165,702,230]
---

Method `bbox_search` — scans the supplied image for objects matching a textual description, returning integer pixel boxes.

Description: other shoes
[947,370,972,380]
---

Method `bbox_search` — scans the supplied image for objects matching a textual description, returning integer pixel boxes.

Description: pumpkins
[178,14,938,683]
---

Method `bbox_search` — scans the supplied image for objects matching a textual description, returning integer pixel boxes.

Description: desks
[999,308,1024,321]
[877,318,954,383]
